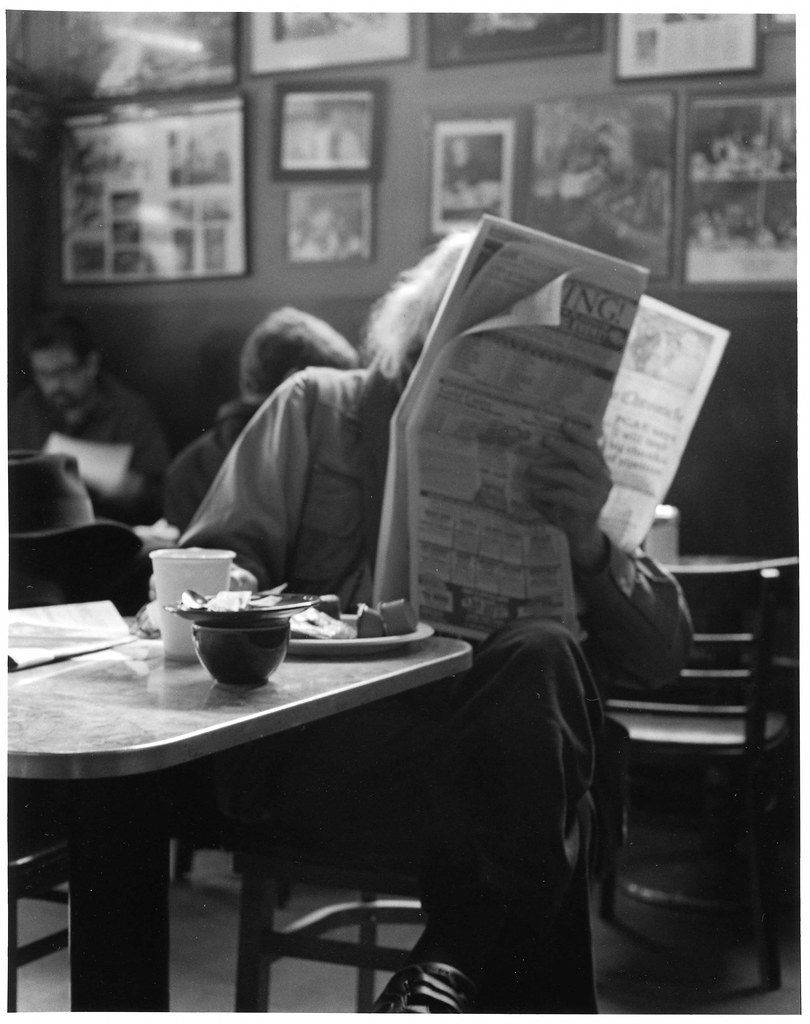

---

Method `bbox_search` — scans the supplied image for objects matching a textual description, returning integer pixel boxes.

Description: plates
[288,613,435,656]
[163,593,321,624]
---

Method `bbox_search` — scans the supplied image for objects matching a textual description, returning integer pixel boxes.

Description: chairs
[10,518,800,1013]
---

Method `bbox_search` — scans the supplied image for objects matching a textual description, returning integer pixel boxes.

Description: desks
[7,620,475,1013]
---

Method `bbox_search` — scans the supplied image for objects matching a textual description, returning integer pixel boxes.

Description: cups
[192,620,291,690]
[644,504,680,566]
[149,547,237,661]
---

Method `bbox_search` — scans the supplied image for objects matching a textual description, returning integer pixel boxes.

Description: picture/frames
[7,13,796,302]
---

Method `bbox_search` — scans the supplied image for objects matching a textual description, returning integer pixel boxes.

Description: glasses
[34,364,79,385]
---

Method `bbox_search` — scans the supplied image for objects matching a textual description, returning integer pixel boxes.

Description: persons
[179,230,694,1013]
[163,307,361,536]
[7,311,172,523]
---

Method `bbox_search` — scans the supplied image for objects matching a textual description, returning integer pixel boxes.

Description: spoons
[182,588,271,610]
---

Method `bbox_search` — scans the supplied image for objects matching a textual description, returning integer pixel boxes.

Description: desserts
[283,606,358,639]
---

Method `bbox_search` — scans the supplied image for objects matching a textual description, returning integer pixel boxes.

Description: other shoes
[369,963,470,1014]
